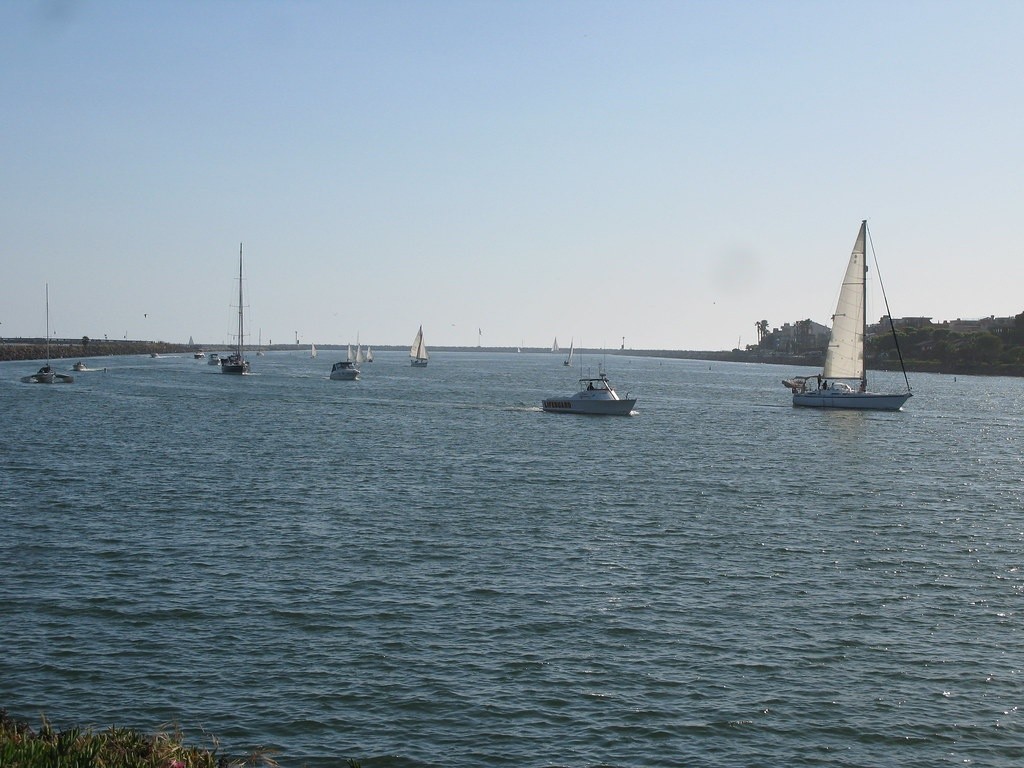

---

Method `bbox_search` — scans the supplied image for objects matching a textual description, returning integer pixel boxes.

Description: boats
[73,361,87,369]
[541,361,639,416]
[329,361,361,381]
[195,347,220,367]
[150,351,160,357]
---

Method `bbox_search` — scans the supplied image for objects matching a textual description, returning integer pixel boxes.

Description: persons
[587,382,594,391]
[822,380,828,390]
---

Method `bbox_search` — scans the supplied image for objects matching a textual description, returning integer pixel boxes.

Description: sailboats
[309,342,318,359]
[782,219,913,411]
[19,282,73,382]
[343,343,374,363]
[562,336,574,366]
[550,338,561,353]
[408,324,429,367]
[256,327,265,356]
[220,243,249,374]
